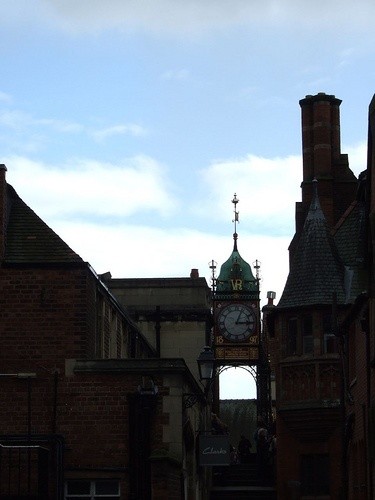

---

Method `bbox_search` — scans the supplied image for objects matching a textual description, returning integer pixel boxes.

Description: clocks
[218,303,256,342]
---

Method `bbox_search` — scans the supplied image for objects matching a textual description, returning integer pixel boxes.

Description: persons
[254,428,276,468]
[236,434,253,466]
